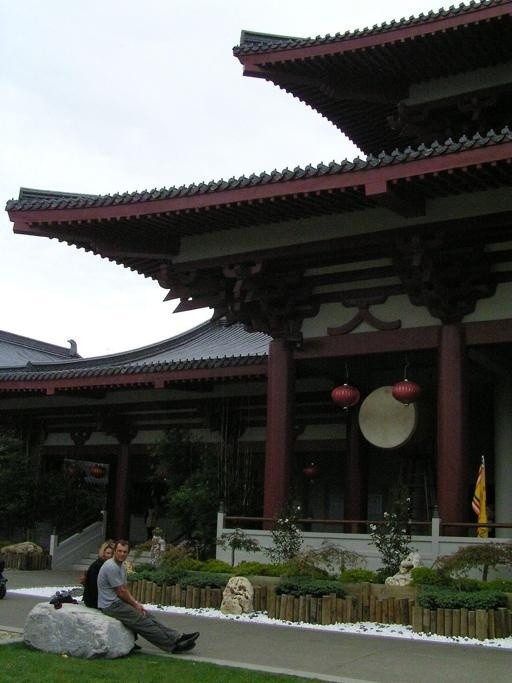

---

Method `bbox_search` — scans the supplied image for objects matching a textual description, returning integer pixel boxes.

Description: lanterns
[392,378,422,407]
[300,464,322,484]
[89,465,109,479]
[330,383,362,412]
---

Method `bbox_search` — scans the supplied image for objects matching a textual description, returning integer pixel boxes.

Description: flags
[471,455,488,538]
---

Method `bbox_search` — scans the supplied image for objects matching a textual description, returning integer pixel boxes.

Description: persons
[96,537,201,653]
[84,539,144,650]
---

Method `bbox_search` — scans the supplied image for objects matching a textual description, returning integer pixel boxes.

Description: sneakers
[177,632,200,647]
[172,641,196,652]
